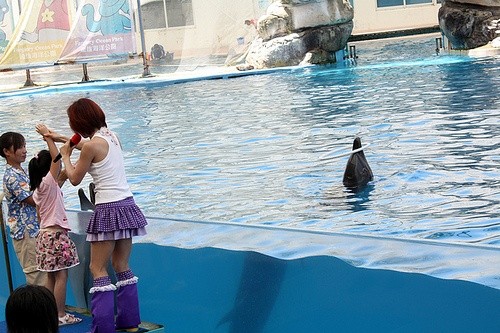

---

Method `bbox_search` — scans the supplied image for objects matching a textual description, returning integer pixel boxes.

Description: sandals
[59,312,83,326]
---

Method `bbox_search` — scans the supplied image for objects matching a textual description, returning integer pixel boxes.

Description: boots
[89,276,117,333]
[116,269,141,332]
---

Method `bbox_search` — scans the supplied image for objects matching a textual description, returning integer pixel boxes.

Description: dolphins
[343,137,374,187]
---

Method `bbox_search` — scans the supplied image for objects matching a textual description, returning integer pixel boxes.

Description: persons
[28,124,83,326]
[5,284,59,333]
[43,98,140,333]
[0,132,48,288]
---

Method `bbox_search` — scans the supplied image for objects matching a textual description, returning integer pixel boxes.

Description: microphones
[53,133,81,163]
